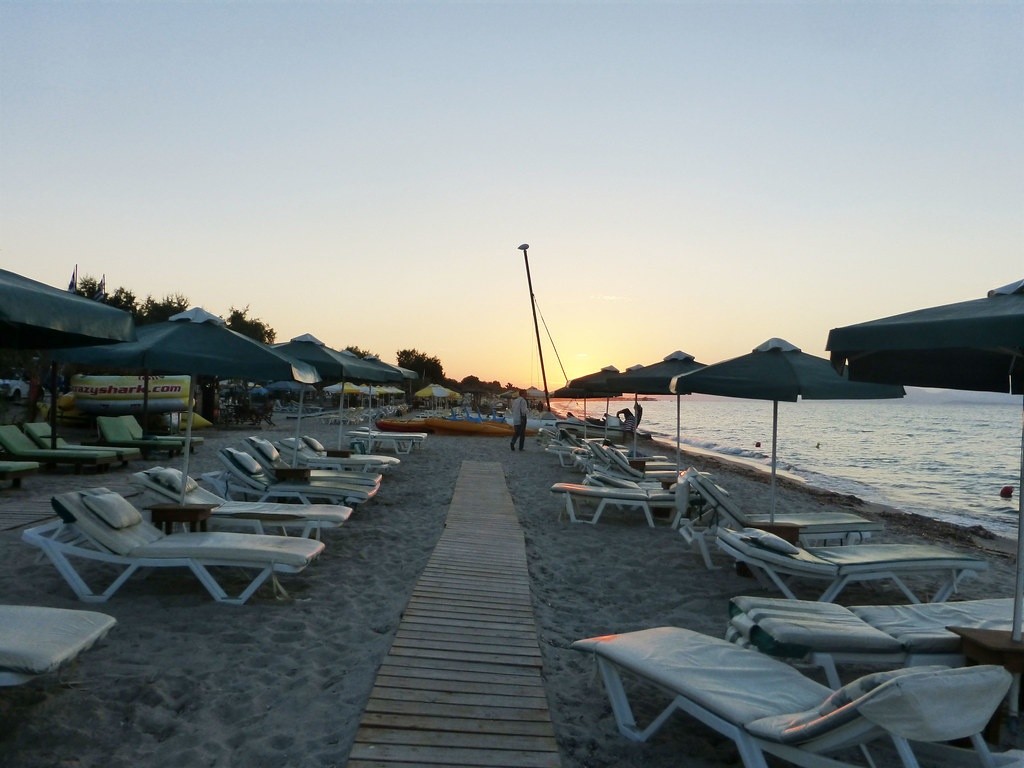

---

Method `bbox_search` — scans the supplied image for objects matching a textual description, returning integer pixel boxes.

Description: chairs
[22,487,326,606]
[0,461,39,489]
[274,436,400,475]
[571,625,1012,768]
[24,422,140,471]
[1,605,115,698]
[724,591,1024,692]
[202,447,377,508]
[129,465,352,559]
[345,426,427,455]
[0,426,117,476]
[535,427,731,527]
[677,469,884,573]
[120,415,205,454]
[715,522,989,607]
[97,418,182,462]
[275,399,462,427]
[241,435,382,487]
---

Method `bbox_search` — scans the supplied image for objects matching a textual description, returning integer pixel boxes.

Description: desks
[322,449,353,457]
[140,501,219,535]
[946,627,1024,744]
[270,468,313,481]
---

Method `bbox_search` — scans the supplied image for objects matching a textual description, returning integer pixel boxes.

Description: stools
[350,441,366,454]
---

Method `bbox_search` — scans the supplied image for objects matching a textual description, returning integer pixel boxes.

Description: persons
[507,390,528,450]
[530,401,543,411]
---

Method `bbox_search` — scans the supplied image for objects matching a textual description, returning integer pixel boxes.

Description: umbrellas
[55,307,459,495]
[552,362,642,442]
[609,350,713,477]
[671,337,907,522]
[824,278,1024,713]
[0,269,140,347]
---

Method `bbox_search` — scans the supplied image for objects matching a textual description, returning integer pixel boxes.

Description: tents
[501,386,547,398]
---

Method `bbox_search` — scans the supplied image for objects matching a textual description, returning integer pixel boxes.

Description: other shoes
[519,448,525,451]
[510,442,515,451]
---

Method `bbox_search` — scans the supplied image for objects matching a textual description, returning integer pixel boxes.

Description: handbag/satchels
[520,415,527,427]
